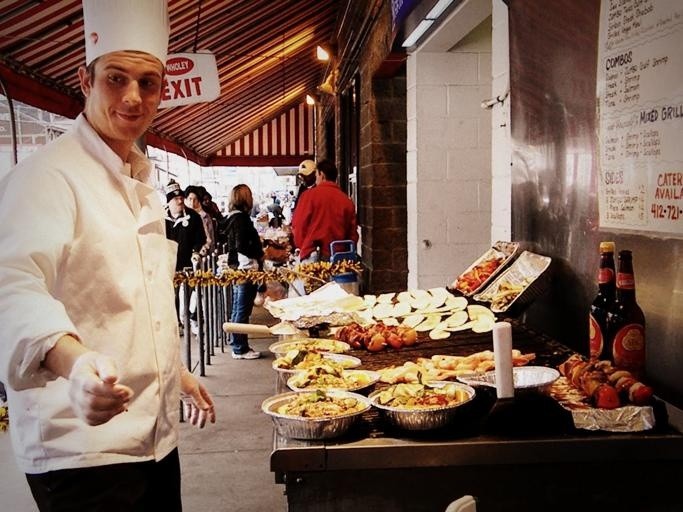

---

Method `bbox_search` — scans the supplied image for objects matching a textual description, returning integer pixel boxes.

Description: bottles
[588,241,616,364]
[606,247,646,383]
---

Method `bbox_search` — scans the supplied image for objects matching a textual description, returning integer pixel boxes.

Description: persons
[0,50,216,511]
[164,159,358,360]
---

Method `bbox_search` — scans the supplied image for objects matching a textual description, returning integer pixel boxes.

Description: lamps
[306,91,320,105]
[316,42,337,60]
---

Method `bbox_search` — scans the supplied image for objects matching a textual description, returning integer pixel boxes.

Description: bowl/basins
[368,363,561,432]
[259,332,384,442]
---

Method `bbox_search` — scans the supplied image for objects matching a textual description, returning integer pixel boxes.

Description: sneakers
[231,348,262,360]
[190,316,199,335]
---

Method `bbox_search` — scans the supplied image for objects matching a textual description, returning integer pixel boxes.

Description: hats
[185,186,206,203]
[82,0,171,68]
[295,160,316,176]
[164,179,185,203]
